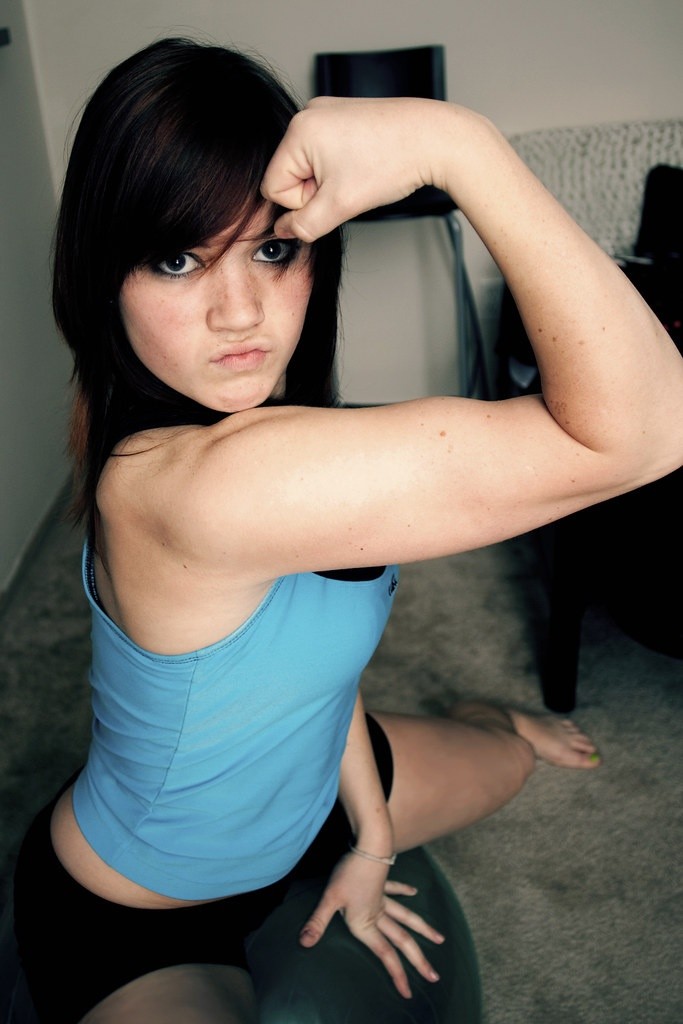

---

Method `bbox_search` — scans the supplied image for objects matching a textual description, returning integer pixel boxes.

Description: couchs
[498,117,683,721]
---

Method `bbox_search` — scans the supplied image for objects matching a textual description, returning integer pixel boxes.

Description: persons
[1,37,683,1024]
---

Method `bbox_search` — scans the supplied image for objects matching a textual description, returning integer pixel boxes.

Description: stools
[310,45,492,401]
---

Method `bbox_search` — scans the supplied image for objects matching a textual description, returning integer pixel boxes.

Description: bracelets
[348,842,397,865]
